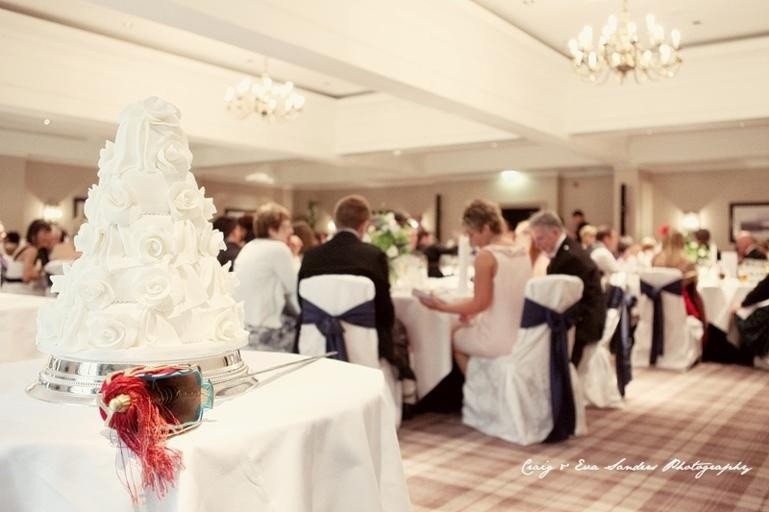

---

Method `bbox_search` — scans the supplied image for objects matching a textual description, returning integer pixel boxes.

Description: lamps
[218,54,309,124]
[561,1,679,85]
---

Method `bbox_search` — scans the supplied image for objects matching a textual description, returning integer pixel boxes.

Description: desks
[0,351,405,512]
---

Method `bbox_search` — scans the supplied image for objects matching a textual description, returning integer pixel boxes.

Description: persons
[0,193,767,379]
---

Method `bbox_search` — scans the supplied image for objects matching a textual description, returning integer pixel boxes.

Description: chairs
[296,225,769,450]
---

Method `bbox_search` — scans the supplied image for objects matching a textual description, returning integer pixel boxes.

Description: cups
[717,263,751,282]
[414,252,428,281]
[438,254,476,279]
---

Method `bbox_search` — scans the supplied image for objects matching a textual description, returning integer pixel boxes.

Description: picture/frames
[729,201,769,244]
